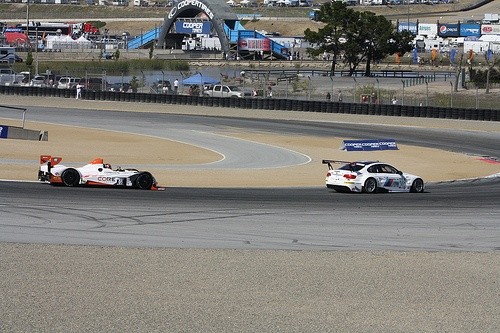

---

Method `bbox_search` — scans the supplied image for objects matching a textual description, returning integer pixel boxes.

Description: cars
[322,159,425,194]
[16,71,250,98]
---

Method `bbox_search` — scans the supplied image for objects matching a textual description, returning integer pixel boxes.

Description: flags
[487,49,493,61]
[432,49,436,61]
[413,48,417,64]
[395,52,401,65]
[467,49,473,63]
[450,49,456,61]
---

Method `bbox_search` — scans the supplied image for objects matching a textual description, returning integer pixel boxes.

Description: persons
[286,50,330,61]
[252,90,258,97]
[391,96,398,104]
[75,83,81,98]
[105,163,121,172]
[173,79,179,92]
[114,49,120,60]
[325,92,331,101]
[338,91,343,102]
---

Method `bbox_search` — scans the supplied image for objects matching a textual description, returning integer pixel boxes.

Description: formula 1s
[39,153,158,191]
